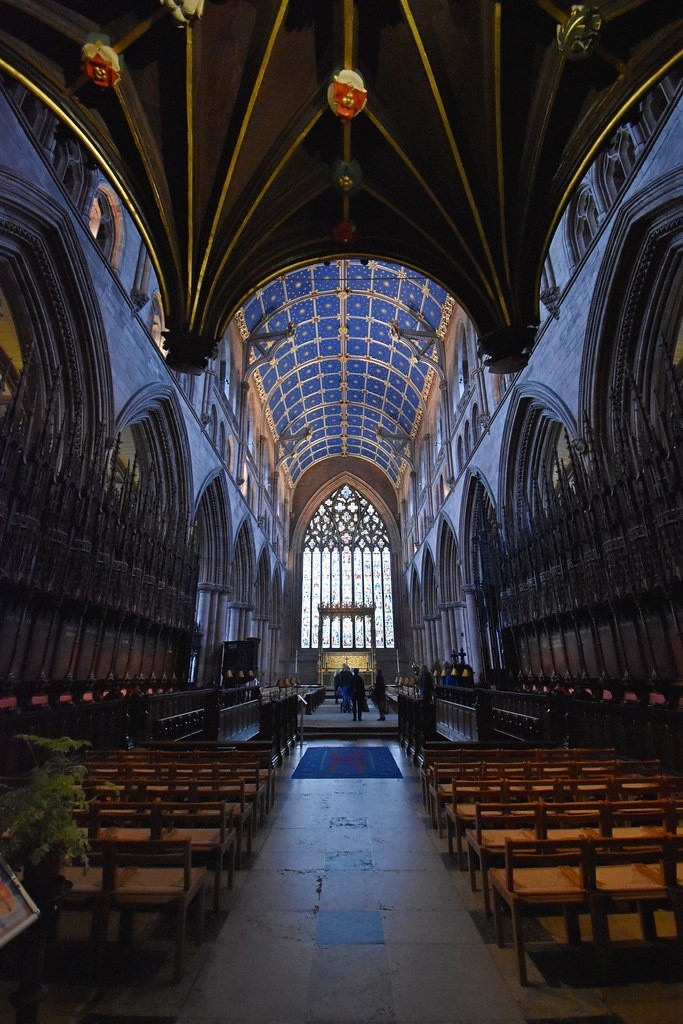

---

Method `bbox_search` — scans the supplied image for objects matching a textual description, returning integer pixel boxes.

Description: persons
[370,667,387,721]
[334,664,365,722]
[415,664,435,702]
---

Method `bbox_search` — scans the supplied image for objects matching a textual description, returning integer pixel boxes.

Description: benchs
[0,749,275,985]
[418,749,683,986]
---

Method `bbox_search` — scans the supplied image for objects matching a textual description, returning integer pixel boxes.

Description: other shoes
[352,717,357,721]
[376,717,386,721]
[359,718,362,722]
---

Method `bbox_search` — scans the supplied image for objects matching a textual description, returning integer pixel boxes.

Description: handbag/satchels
[363,699,369,712]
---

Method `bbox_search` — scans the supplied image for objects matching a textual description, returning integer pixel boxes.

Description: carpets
[291,747,404,778]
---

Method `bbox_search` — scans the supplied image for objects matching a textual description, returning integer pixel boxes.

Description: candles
[396,649,400,674]
[295,650,297,673]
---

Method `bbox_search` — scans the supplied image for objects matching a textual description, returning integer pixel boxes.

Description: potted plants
[0,732,121,894]
